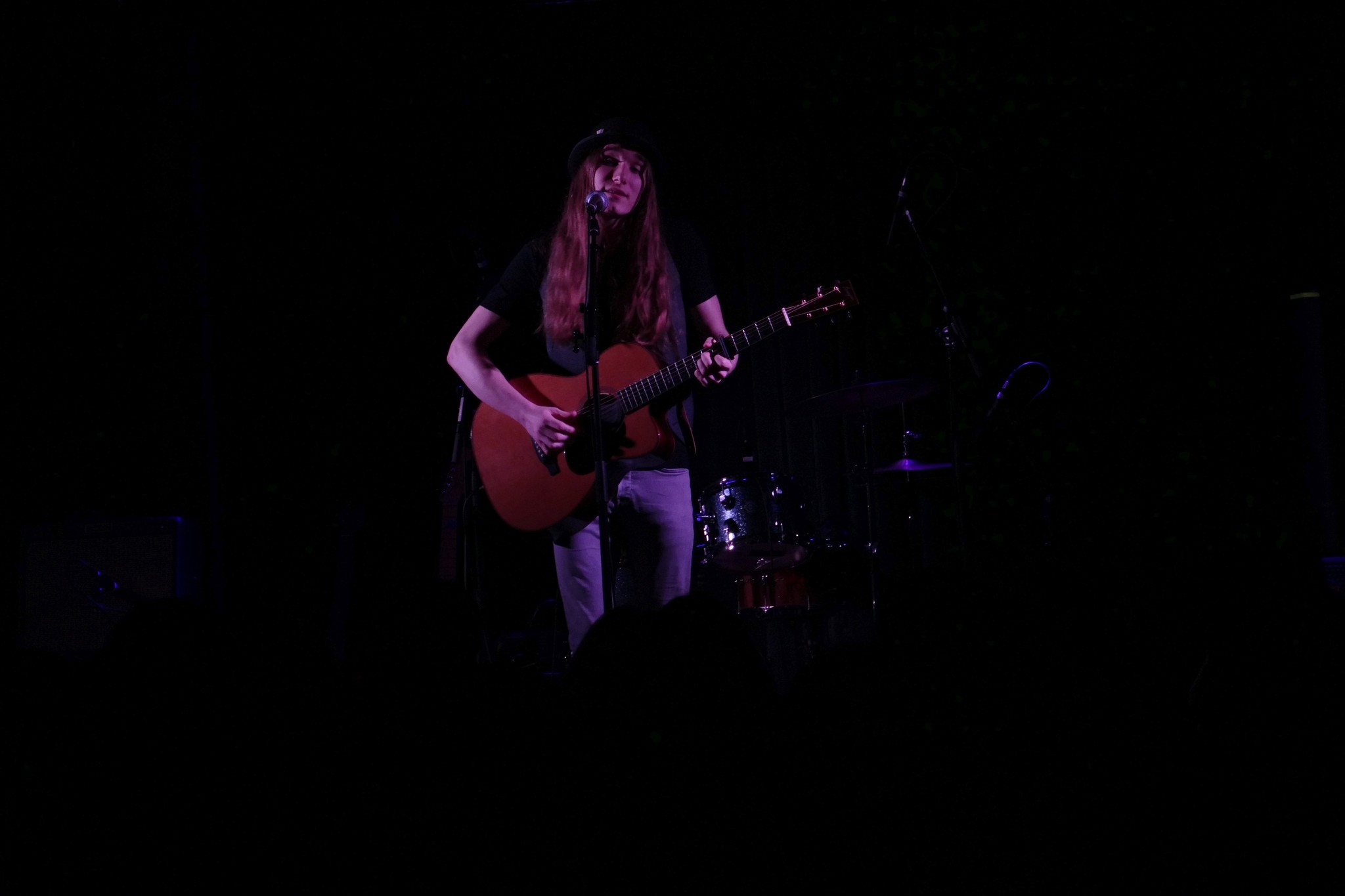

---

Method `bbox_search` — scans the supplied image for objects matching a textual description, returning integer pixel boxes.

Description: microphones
[585,190,608,214]
[888,179,907,246]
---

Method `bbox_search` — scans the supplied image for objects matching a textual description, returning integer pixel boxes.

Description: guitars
[470,272,862,533]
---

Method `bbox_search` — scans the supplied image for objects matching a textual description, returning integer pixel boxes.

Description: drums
[737,566,814,613]
[695,470,809,573]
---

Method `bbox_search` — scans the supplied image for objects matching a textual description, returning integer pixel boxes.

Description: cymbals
[871,457,957,475]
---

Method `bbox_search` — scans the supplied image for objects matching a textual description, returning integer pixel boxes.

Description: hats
[567,118,659,179]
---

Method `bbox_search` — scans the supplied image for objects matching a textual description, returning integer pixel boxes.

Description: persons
[446,115,739,655]
